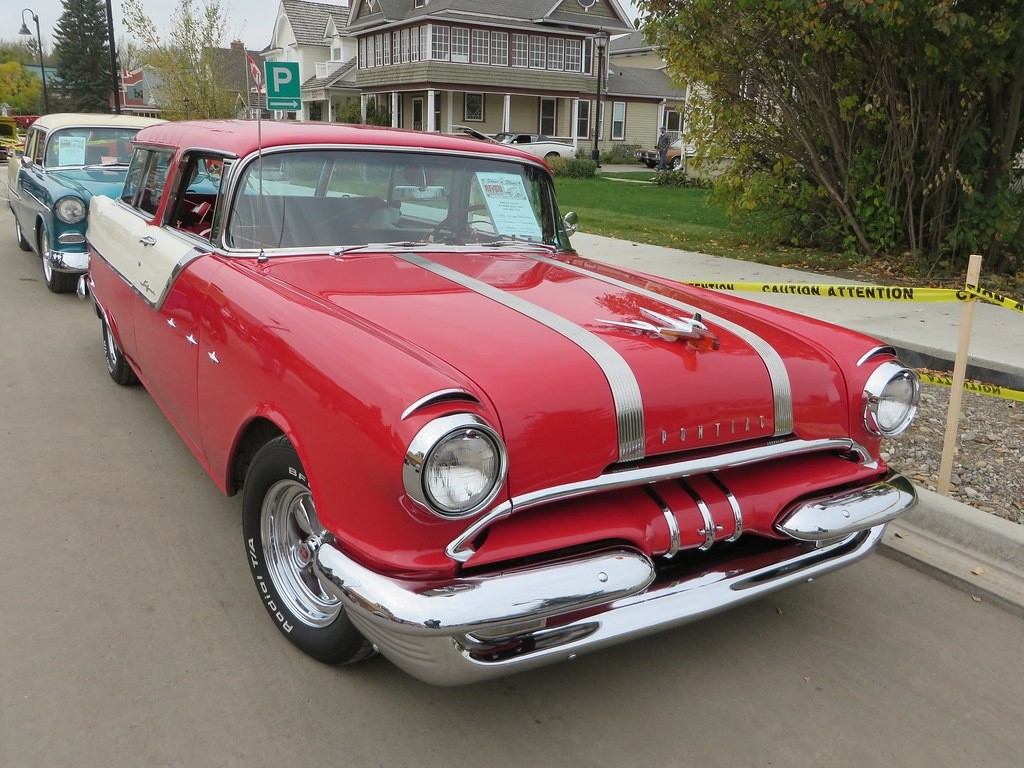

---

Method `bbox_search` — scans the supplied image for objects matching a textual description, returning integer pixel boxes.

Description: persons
[656,126,671,171]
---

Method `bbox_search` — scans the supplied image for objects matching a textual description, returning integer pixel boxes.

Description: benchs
[163,194,400,241]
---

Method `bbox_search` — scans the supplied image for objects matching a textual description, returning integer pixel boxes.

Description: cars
[446,125,577,160]
[7,113,177,294]
[635,131,688,169]
[76,120,919,689]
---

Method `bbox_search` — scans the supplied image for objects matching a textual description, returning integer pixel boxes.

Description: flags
[247,54,262,95]
[123,67,135,78]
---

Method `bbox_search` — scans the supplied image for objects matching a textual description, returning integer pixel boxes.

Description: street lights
[592,26,609,76]
[18,7,45,67]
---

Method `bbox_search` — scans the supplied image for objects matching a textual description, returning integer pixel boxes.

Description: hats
[659,126,666,129]
